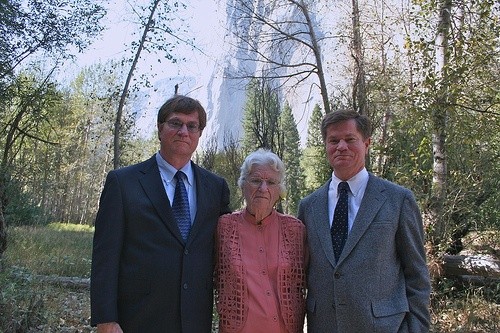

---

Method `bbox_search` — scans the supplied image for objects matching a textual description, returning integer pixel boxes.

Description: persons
[297,109,431,333]
[90,96,232,333]
[216,149,309,332]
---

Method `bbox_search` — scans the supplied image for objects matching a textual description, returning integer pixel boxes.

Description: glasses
[162,119,199,133]
[245,177,278,188]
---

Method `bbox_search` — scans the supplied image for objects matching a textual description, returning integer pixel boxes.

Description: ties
[330,182,350,265]
[172,171,191,245]
